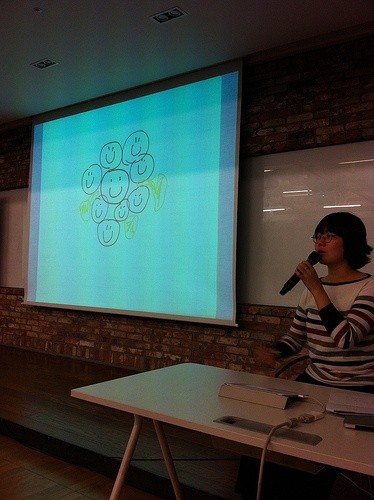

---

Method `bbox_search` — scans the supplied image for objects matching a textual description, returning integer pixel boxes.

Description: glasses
[311,231,338,244]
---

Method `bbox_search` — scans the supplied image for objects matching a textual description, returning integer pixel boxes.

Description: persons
[235,212,374,500]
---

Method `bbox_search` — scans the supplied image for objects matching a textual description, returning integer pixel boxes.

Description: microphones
[280,250,322,296]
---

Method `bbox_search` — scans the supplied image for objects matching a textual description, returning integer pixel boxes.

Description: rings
[305,267,309,271]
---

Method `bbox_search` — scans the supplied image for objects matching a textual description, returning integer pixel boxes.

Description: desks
[71,361,374,500]
